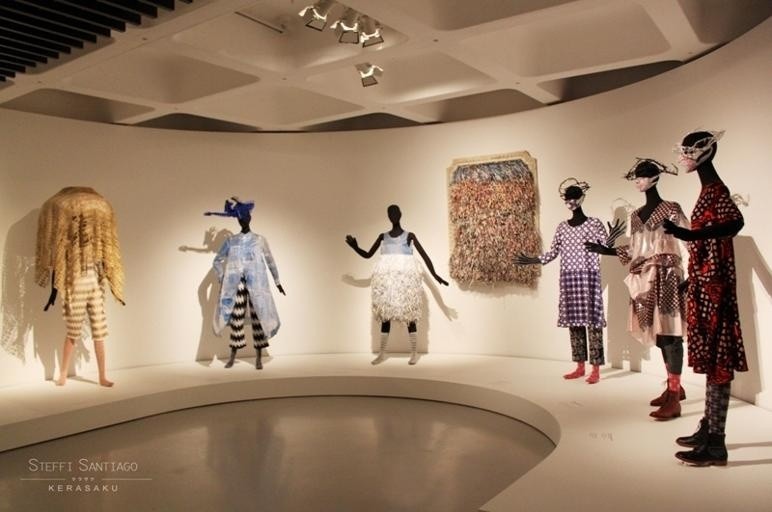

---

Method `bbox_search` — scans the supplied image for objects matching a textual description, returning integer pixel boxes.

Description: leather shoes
[649,385,686,418]
[676,420,728,465]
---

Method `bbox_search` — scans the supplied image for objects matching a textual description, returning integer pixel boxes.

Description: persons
[33,184,127,387]
[584,156,693,419]
[657,127,747,467]
[344,203,453,365]
[511,177,629,384]
[203,197,289,370]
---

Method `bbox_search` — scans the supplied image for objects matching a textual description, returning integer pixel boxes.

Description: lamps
[298,0,385,49]
[354,62,384,88]
